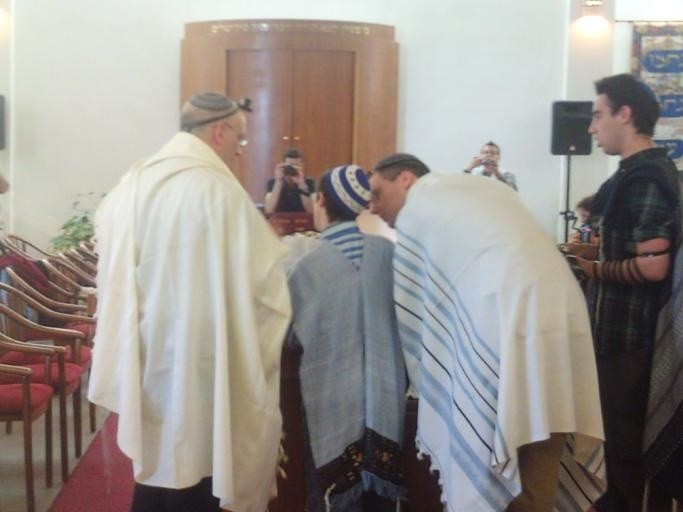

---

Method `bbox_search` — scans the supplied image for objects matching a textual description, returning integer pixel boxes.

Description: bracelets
[464,169,471,173]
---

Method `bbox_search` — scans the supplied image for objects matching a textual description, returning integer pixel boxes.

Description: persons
[86,91,294,512]
[366,153,608,511]
[264,147,316,215]
[287,164,407,511]
[464,140,517,192]
[572,195,595,246]
[566,73,683,512]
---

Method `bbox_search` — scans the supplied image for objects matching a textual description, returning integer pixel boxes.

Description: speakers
[552,101,594,155]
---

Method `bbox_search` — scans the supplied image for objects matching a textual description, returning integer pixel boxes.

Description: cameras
[481,159,492,166]
[283,166,298,176]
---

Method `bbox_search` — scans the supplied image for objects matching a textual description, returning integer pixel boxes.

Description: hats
[326,164,372,217]
[181,91,237,129]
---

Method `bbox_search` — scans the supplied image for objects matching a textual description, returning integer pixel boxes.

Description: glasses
[224,122,247,148]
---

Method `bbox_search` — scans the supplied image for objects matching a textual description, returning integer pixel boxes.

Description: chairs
[1,234,97,512]
